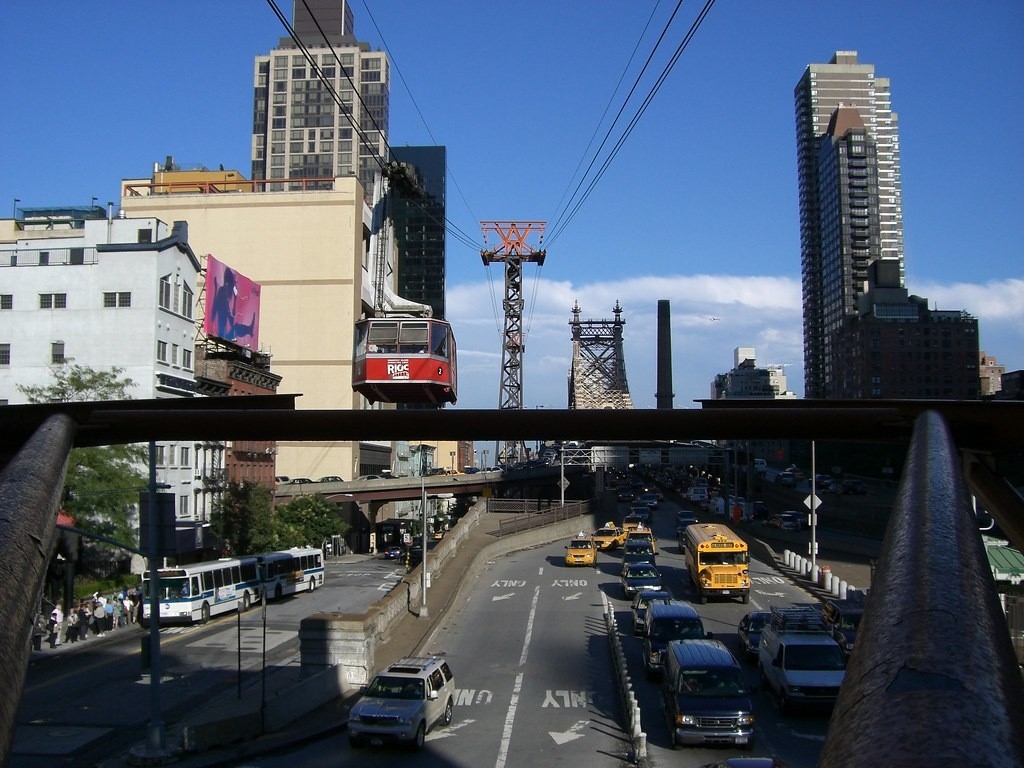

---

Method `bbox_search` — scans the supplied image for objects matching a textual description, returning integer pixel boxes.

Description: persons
[733,504,741,526]
[32,579,194,651]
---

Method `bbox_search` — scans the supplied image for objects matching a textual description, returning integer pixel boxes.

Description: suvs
[621,541,655,569]
[346,656,456,751]
[755,604,849,719]
[658,639,758,753]
[642,603,713,674]
[823,597,866,662]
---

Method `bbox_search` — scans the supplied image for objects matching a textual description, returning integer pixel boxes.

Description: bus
[683,524,754,605]
[140,550,325,627]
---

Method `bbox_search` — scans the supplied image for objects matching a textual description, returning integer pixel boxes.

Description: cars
[737,611,772,668]
[564,529,598,568]
[625,520,655,552]
[620,562,665,601]
[434,531,445,539]
[590,442,871,553]
[630,590,671,635]
[276,466,503,487]
[384,547,403,559]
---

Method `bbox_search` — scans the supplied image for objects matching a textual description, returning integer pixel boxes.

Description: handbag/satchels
[104,612,108,619]
[131,605,134,612]
[123,606,128,614]
[46,624,53,631]
[72,621,81,628]
[89,616,94,624]
[33,627,47,636]
[53,624,60,633]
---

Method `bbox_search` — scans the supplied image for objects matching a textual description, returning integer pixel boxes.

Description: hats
[51,613,57,616]
[224,267,237,283]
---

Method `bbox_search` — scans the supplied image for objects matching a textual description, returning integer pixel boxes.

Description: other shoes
[94,629,116,637]
[55,644,61,646]
[63,634,89,643]
[50,646,57,649]
